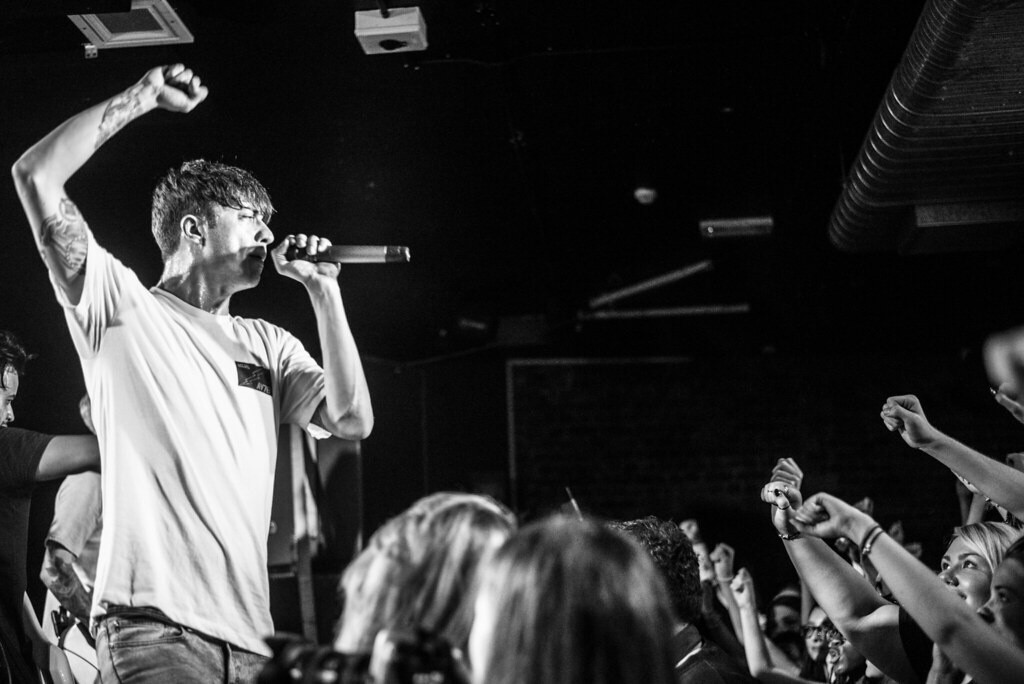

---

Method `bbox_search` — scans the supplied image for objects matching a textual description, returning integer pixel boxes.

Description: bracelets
[777,532,801,540]
[860,525,882,552]
[716,575,733,582]
[862,530,887,554]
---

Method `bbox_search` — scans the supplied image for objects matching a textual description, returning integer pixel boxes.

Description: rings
[777,504,791,510]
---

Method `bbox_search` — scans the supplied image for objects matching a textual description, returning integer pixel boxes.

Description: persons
[335,334,1024,684]
[10,63,375,684]
[0,336,105,684]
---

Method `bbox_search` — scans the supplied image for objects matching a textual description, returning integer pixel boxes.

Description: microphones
[284,243,412,263]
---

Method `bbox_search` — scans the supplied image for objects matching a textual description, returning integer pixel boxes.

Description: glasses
[826,630,846,641]
[800,626,826,640]
